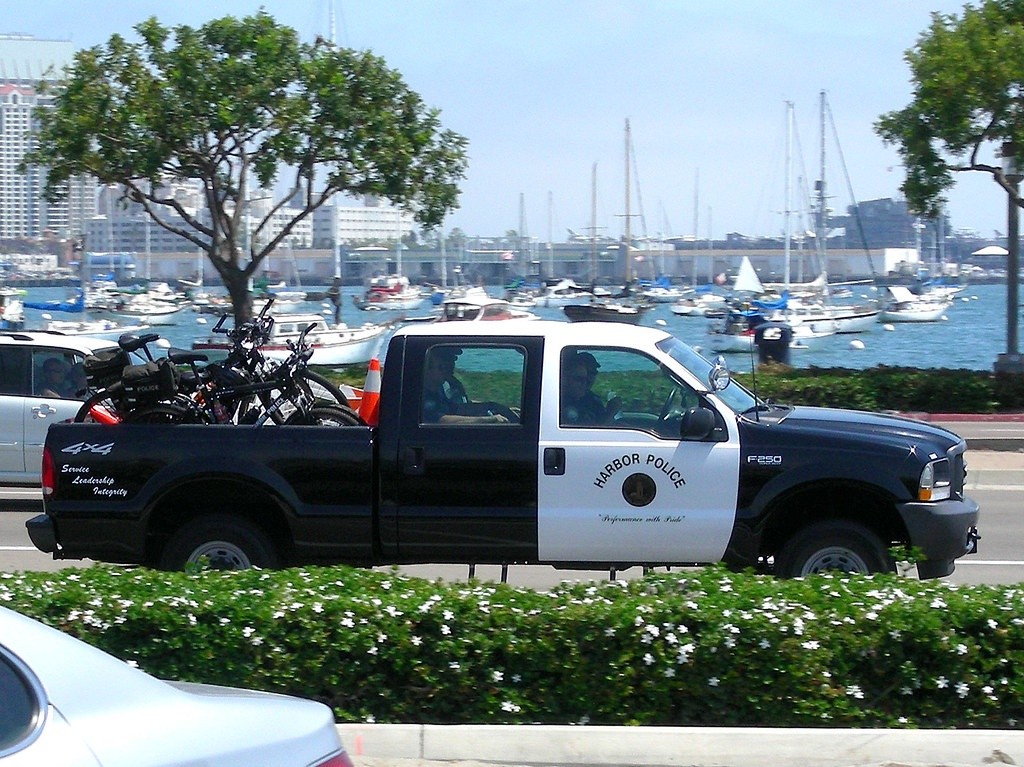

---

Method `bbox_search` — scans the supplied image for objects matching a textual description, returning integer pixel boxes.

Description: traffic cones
[359,358,382,426]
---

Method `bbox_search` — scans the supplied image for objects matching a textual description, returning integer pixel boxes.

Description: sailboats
[501,94,967,351]
[0,186,306,325]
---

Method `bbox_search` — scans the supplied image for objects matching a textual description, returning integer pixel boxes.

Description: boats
[354,198,541,328]
[191,312,387,367]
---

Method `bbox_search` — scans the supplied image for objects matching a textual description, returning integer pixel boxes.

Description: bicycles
[74,298,359,427]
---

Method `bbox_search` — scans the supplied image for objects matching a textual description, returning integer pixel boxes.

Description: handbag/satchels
[120,359,178,398]
[83,345,133,389]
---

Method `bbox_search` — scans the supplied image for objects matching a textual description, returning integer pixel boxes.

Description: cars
[0,605,353,767]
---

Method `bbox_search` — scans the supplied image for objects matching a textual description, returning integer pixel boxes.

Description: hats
[579,352,600,368]
[431,345,462,354]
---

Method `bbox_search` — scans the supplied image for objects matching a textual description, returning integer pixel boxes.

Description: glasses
[568,373,590,385]
[444,355,458,361]
[46,368,64,375]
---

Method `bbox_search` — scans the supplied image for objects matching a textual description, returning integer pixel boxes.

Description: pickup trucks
[24,322,981,580]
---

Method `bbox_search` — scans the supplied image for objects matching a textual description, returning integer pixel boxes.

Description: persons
[55,362,87,397]
[422,347,521,424]
[575,352,622,419]
[38,358,65,398]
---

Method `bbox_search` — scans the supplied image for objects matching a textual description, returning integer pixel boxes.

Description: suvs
[0,330,132,488]
